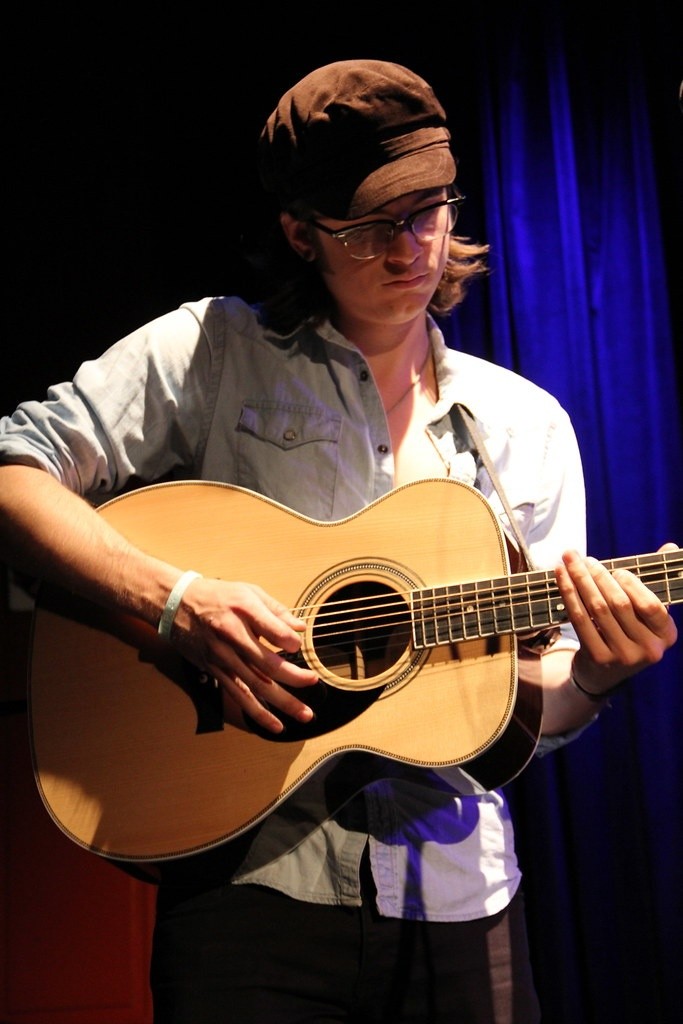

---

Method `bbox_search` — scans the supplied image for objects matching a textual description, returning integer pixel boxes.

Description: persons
[0,60,679,1024]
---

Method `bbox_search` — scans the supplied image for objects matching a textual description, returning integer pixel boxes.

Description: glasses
[290,183,465,260]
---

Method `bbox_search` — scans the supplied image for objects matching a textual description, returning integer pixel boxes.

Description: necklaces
[386,342,431,416]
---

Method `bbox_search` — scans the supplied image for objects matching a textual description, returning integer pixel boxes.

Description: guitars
[24,476,683,892]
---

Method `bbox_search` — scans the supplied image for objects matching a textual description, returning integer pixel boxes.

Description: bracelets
[568,662,618,703]
[158,571,202,643]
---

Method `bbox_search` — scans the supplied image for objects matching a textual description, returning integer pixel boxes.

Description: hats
[258,58,457,220]
